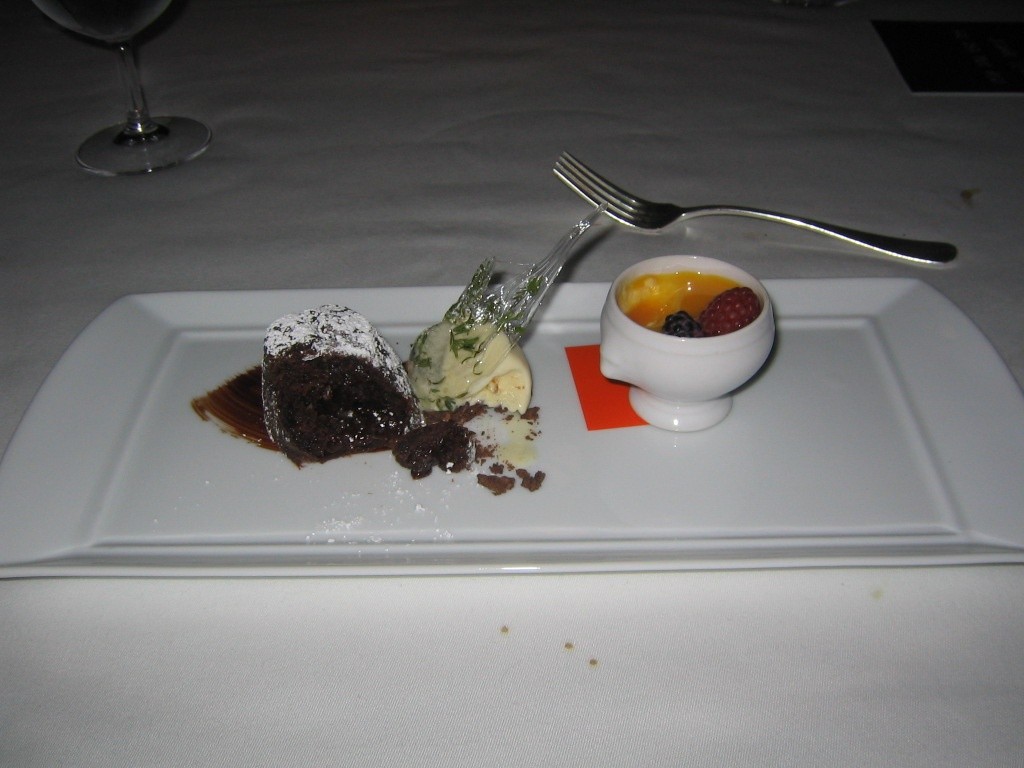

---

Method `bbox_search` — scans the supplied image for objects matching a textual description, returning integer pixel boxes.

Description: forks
[552,150,958,266]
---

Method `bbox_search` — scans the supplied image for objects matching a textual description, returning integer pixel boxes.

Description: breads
[262,303,545,494]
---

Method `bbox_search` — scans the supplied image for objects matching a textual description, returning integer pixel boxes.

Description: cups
[599,254,776,431]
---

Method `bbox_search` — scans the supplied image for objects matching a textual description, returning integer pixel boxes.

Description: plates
[0,278,1023,571]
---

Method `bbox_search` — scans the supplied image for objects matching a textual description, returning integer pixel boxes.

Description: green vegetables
[410,258,543,412]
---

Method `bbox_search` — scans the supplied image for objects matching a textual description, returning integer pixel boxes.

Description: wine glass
[32,0,212,177]
